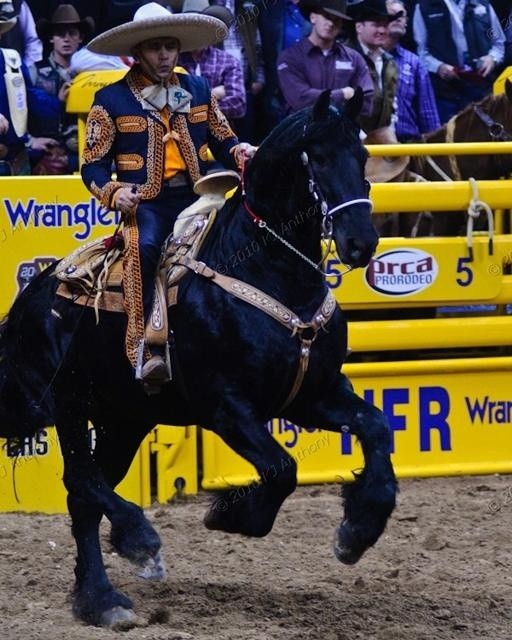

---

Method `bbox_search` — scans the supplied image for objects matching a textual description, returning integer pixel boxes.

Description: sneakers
[142,356,166,395]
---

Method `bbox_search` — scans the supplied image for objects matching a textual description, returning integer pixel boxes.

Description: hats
[297,0,353,22]
[181,4,235,27]
[35,4,95,39]
[87,3,229,54]
[347,0,402,22]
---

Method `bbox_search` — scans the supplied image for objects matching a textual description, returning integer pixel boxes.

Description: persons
[78,36,261,380]
[0,2,512,180]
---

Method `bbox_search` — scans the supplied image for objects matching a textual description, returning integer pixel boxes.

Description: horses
[0,86,400,631]
[373,168,428,230]
[414,78,511,236]
